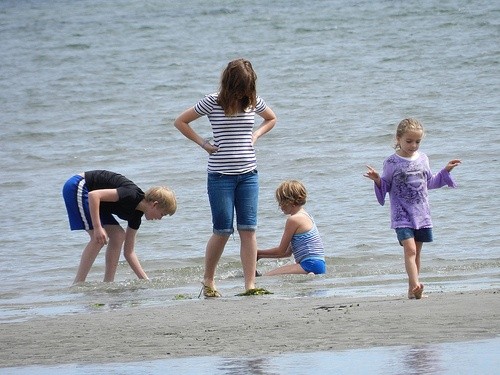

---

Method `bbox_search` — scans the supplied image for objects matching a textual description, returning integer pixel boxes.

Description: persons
[173,58,276,297]
[364,117,462,299]
[256,181,326,275]
[62,171,176,282]
